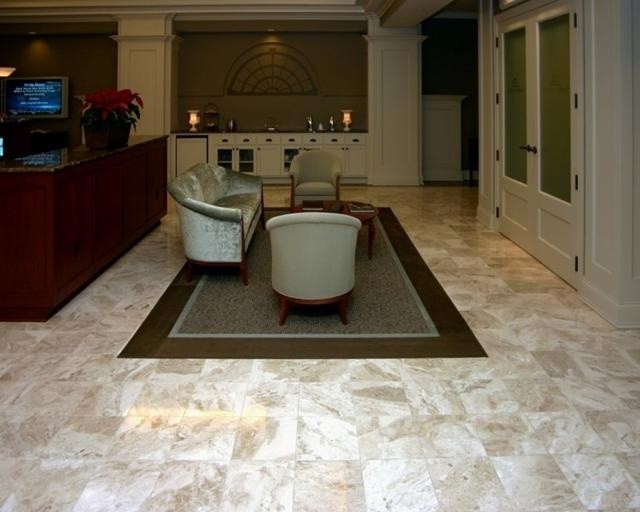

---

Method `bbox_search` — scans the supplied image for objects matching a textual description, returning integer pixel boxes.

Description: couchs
[265,212,362,325]
[167,162,265,286]
[288,150,343,212]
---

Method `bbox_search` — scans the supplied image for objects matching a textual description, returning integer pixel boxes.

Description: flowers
[79,86,144,133]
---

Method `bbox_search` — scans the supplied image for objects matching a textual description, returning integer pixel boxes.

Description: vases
[84,121,132,149]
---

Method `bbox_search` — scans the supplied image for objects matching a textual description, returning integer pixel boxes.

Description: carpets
[167,210,441,338]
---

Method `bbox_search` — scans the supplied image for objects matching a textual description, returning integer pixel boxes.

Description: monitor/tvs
[2,76,70,119]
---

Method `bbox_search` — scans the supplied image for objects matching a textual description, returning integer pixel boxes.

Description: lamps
[186,109,201,132]
[340,109,353,132]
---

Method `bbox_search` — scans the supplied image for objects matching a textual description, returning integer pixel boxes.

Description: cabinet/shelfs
[256,133,281,184]
[324,133,368,185]
[422,94,468,181]
[170,133,214,180]
[213,133,256,176]
[281,133,324,185]
